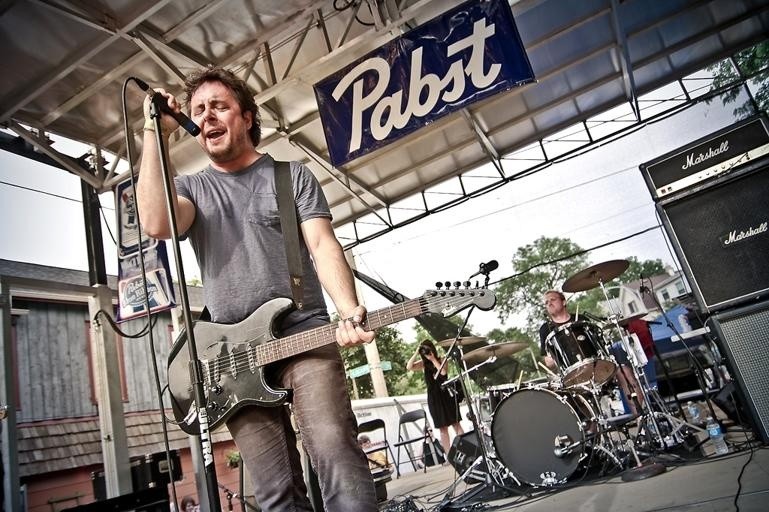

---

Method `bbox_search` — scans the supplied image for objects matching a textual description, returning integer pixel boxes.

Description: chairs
[396,409,444,478]
[358,419,400,476]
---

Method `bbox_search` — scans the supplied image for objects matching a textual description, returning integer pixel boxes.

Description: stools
[606,414,666,485]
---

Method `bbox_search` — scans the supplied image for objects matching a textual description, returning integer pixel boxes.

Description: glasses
[360,441,366,445]
[425,430,434,433]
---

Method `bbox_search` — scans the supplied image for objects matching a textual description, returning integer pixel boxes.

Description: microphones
[134,78,201,137]
[640,274,644,298]
[478,259,499,274]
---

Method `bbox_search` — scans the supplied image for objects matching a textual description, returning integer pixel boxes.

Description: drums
[490,379,605,489]
[544,319,617,394]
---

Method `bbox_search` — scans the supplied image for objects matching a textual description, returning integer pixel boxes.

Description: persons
[181,494,200,512]
[357,435,394,475]
[537,290,645,416]
[416,424,452,468]
[405,338,467,466]
[136,66,377,512]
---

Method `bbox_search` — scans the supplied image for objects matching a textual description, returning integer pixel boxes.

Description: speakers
[655,158,769,315]
[708,299,769,440]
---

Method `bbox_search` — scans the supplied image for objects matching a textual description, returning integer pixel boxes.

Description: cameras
[418,346,430,355]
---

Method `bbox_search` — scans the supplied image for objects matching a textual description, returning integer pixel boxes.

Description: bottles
[687,401,706,425]
[706,417,729,455]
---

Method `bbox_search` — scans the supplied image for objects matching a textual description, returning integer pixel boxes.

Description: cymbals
[602,311,647,330]
[434,337,487,346]
[461,340,530,361]
[562,260,629,292]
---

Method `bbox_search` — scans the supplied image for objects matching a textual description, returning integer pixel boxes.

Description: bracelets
[143,121,172,138]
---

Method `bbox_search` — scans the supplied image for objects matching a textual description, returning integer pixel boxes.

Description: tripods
[434,278,533,506]
[644,287,748,452]
[547,277,699,476]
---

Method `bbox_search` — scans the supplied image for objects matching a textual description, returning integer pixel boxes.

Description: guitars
[167,280,496,435]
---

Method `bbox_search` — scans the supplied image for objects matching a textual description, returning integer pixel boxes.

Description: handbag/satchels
[442,377,465,403]
